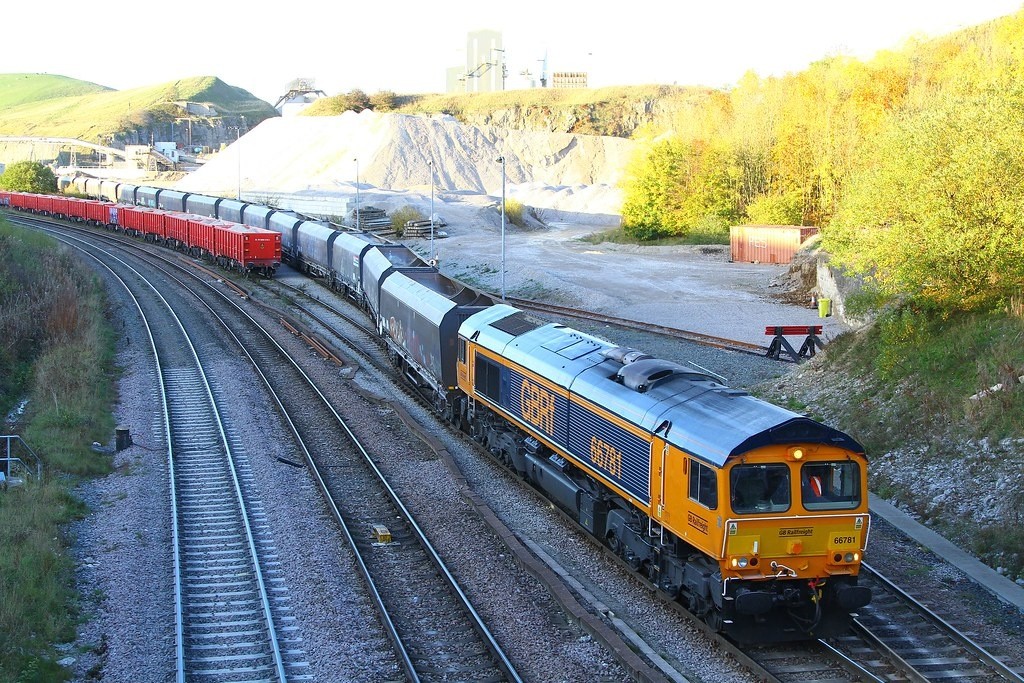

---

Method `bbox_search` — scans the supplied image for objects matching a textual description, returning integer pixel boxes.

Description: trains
[56,176,874,645]
[0,191,284,277]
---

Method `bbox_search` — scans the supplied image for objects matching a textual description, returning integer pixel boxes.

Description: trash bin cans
[115,429,129,450]
[818,298,831,318]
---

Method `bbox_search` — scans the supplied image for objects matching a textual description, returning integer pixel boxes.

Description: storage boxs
[730,224,817,264]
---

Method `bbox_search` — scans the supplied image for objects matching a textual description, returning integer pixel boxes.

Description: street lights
[353,158,360,233]
[493,155,506,302]
[427,159,435,259]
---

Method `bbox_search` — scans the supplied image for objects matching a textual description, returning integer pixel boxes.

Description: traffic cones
[809,292,819,310]
